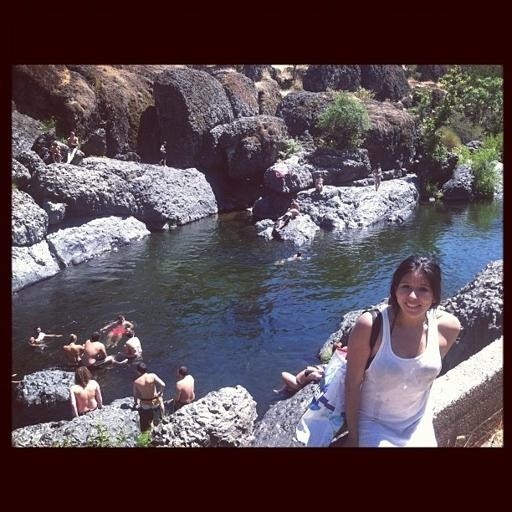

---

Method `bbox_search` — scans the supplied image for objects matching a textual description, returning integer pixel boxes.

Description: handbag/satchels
[292,306,384,447]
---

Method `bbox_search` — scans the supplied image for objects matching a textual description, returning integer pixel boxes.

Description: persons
[372,162,383,192]
[40,131,78,164]
[63,333,85,353]
[294,251,302,260]
[277,199,300,228]
[82,332,128,368]
[100,316,133,349]
[273,364,324,395]
[158,141,168,167]
[316,173,323,194]
[133,362,165,411]
[343,254,462,447]
[28,327,62,348]
[125,330,143,360]
[11,373,23,384]
[173,365,195,410]
[69,366,102,417]
